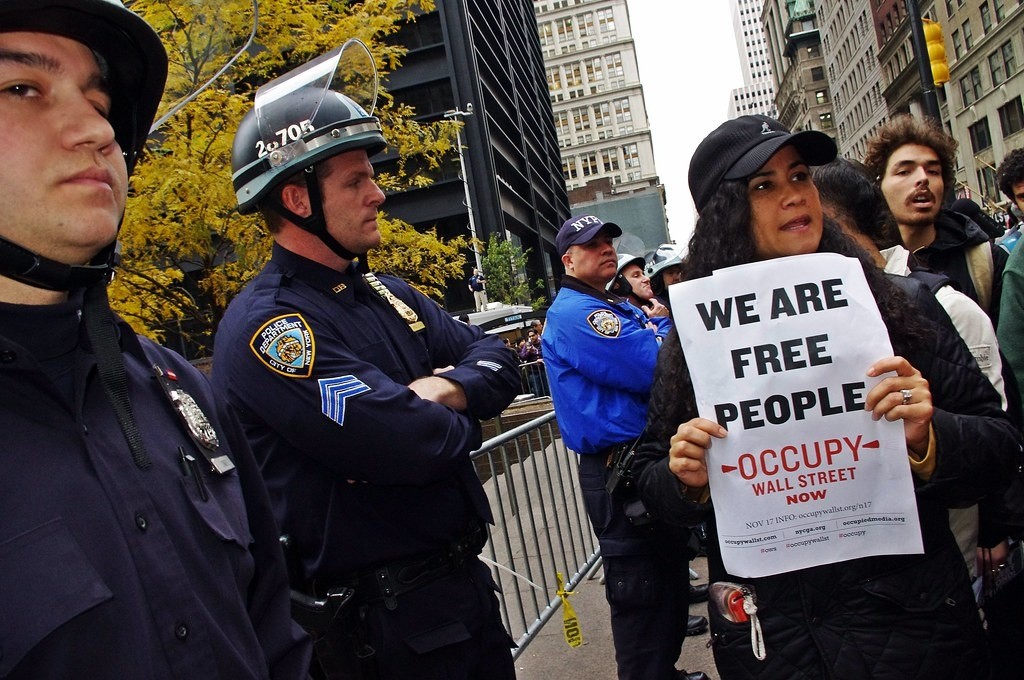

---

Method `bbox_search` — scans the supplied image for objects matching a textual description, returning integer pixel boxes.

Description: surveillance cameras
[466,103,474,112]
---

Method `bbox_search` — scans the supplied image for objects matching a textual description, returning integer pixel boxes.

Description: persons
[0,1,315,680]
[469,269,488,311]
[458,314,471,326]
[541,216,709,680]
[502,319,549,397]
[606,113,1024,679]
[209,38,522,679]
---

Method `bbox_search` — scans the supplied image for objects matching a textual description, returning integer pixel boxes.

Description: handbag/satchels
[707,581,767,661]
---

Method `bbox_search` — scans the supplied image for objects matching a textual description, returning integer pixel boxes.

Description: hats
[555,215,622,257]
[688,114,838,212]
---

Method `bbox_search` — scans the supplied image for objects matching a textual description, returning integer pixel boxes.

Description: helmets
[0,0,168,179]
[606,254,646,291]
[644,245,684,278]
[230,87,389,215]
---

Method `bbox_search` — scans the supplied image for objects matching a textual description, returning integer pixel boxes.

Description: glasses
[528,335,535,337]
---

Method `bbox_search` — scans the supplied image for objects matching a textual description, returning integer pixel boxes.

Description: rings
[999,565,1004,567]
[900,390,912,404]
[992,569,1000,572]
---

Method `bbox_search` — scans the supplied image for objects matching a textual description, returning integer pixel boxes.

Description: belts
[343,549,472,602]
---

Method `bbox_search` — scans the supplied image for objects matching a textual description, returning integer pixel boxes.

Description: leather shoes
[679,669,708,680]
[690,584,708,602]
[686,616,708,636]
